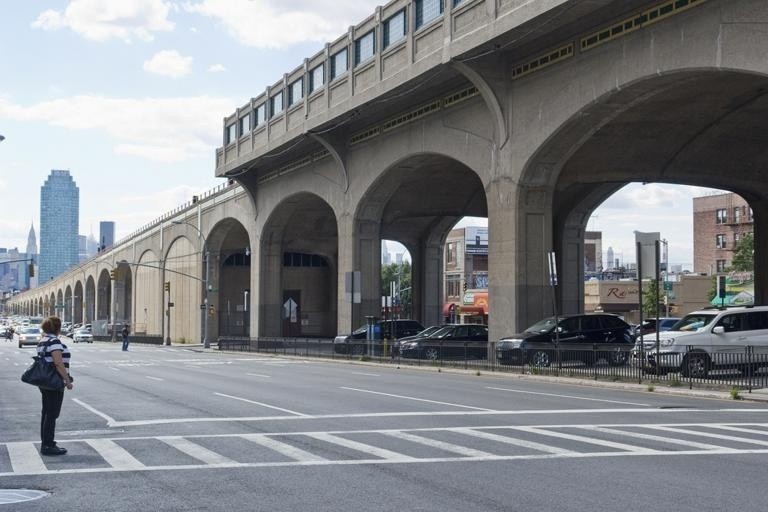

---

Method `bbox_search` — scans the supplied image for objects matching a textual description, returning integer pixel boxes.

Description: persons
[36,316,73,455]
[121,324,130,352]
[690,318,704,331]
[5,330,13,341]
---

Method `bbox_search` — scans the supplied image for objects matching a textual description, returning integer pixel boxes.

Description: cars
[399,323,489,362]
[390,326,443,356]
[104,322,125,332]
[72,328,93,343]
[0,313,91,338]
[631,316,685,337]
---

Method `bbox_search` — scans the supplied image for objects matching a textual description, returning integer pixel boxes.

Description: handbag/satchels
[20,337,64,391]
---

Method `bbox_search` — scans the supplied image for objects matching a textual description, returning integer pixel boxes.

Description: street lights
[92,259,117,343]
[172,220,210,350]
[65,262,86,285]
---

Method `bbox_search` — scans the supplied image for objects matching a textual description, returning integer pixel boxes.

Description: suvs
[494,308,633,369]
[630,301,767,380]
[17,327,42,348]
[330,317,425,357]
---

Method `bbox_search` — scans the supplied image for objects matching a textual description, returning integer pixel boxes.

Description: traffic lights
[164,281,170,292]
[462,282,466,291]
[109,268,118,282]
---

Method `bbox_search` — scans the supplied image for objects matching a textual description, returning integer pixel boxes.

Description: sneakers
[40,443,67,455]
